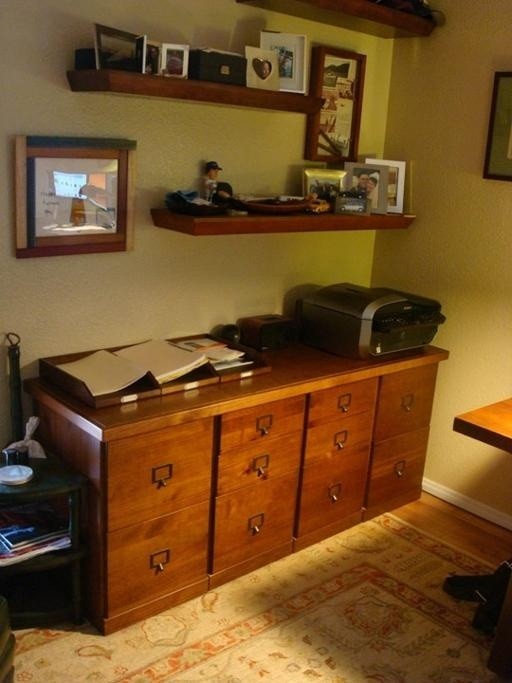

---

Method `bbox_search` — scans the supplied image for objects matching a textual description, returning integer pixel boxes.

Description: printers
[302,283,446,361]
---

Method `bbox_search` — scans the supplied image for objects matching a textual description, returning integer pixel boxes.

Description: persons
[366,178,379,207]
[350,172,369,194]
[203,161,222,204]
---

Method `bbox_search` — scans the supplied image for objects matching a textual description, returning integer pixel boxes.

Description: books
[0,501,72,567]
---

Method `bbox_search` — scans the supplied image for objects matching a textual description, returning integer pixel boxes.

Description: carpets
[9,507,506,683]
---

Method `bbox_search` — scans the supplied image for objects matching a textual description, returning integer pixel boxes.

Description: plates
[243,195,313,212]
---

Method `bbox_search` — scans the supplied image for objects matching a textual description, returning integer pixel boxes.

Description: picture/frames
[10,130,138,259]
[88,15,307,94]
[304,40,370,164]
[299,155,415,219]
[476,69,512,184]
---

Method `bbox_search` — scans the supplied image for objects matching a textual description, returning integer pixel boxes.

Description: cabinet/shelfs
[21,333,454,641]
[1,446,96,634]
[63,0,444,237]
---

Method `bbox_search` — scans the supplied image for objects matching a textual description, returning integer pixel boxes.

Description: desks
[451,387,512,681]
